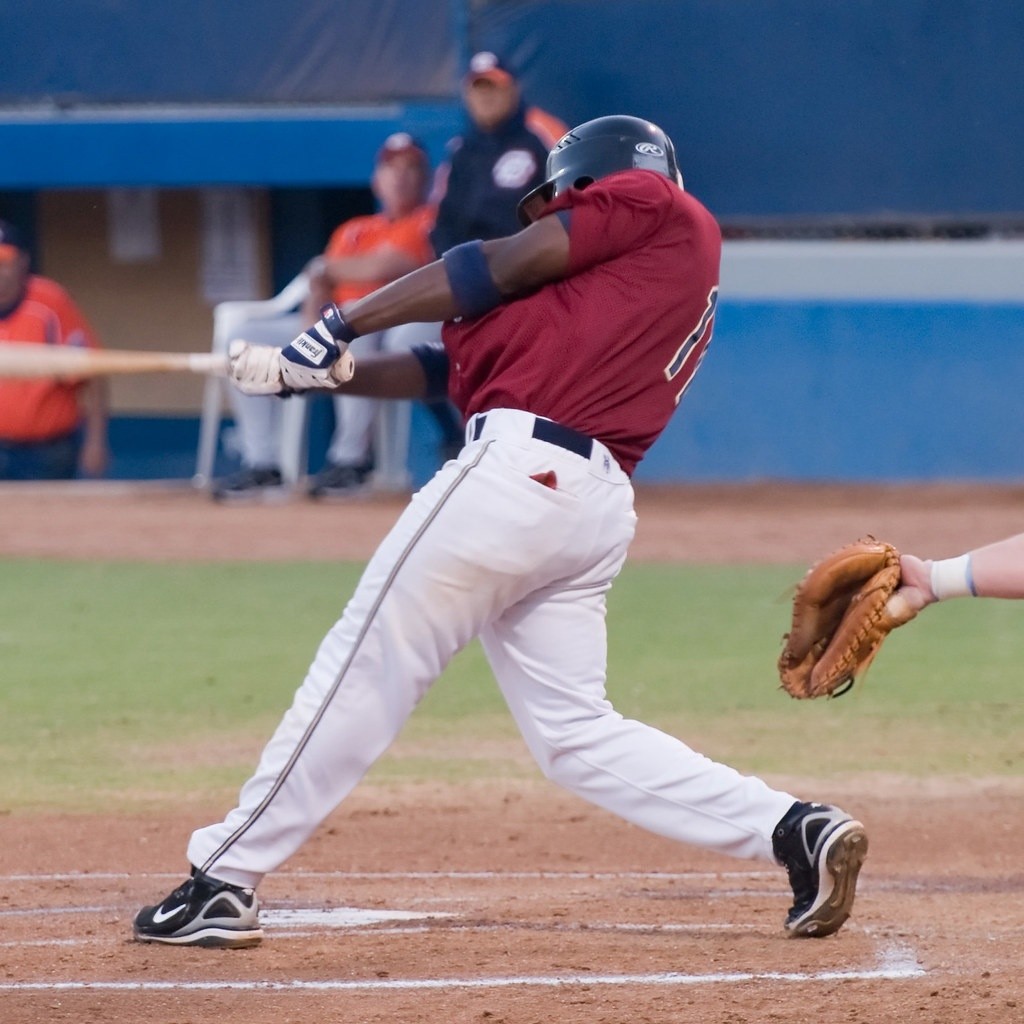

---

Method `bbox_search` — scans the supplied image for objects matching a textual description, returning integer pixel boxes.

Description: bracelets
[928,553,977,602]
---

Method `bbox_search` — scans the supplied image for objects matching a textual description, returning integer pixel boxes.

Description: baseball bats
[0,344,358,384]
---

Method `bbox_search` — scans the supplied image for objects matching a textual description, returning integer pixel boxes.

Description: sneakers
[306,460,377,504]
[207,463,286,508]
[131,862,264,949]
[773,801,869,937]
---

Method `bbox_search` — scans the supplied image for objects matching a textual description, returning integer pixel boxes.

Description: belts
[472,415,593,460]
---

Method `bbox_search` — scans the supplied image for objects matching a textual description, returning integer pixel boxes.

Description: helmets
[516,114,684,228]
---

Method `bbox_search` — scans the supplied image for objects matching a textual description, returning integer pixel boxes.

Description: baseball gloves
[778,543,905,701]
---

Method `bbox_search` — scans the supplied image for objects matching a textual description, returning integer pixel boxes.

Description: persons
[212,130,445,507]
[885,533,1024,626]
[0,220,111,480]
[131,112,871,948]
[429,50,573,262]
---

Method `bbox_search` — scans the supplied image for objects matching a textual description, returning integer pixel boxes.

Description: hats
[0,220,28,264]
[455,47,518,91]
[373,131,432,169]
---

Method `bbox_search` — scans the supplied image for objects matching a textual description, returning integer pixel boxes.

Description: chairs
[191,255,441,502]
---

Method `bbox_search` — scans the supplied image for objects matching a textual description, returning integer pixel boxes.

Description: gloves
[279,301,360,390]
[228,339,307,399]
